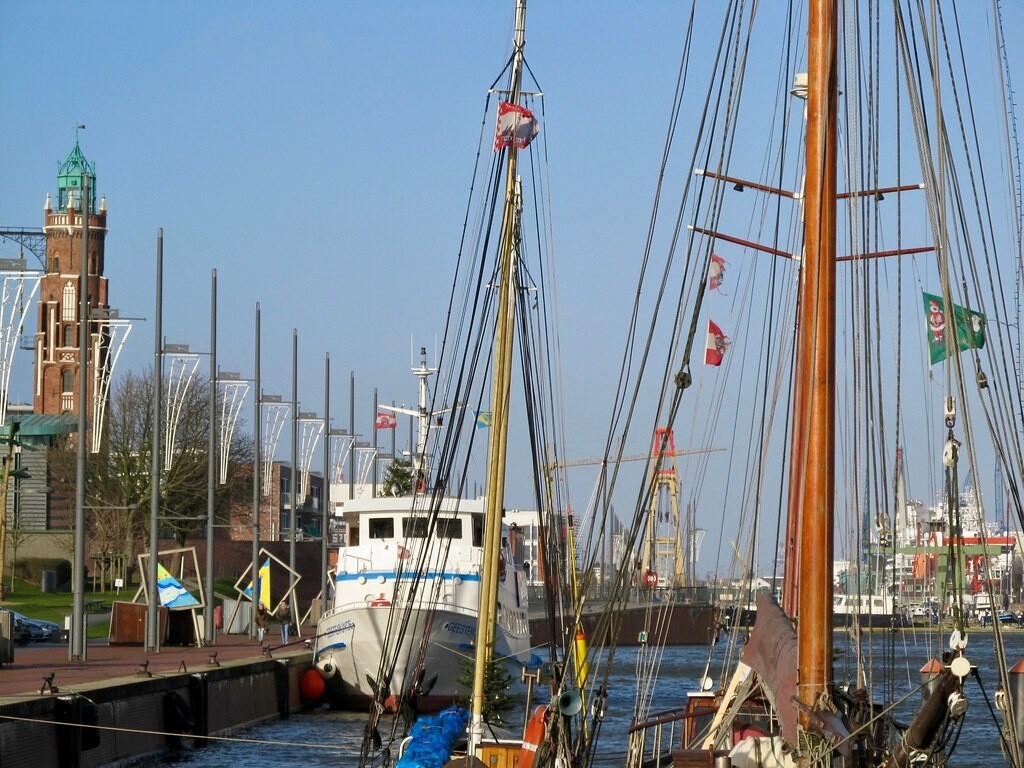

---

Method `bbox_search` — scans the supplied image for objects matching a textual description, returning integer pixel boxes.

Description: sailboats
[578,1,1024,767]
[333,1,608,767]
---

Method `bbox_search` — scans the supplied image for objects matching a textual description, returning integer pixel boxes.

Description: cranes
[535,444,728,524]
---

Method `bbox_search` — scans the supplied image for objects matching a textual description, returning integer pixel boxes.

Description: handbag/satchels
[289,623,298,637]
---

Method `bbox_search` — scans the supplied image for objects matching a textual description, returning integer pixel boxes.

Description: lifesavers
[413,479,426,493]
[644,571,658,586]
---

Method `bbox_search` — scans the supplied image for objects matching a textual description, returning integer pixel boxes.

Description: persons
[276,601,292,646]
[255,604,268,647]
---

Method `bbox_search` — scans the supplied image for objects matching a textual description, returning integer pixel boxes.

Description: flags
[923,296,987,365]
[705,319,730,368]
[473,411,493,430]
[493,103,542,151]
[375,413,397,429]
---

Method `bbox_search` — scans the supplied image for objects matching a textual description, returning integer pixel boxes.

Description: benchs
[71,599,105,611]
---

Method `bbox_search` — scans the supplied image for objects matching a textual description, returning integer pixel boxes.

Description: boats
[311,330,534,702]
[519,427,721,649]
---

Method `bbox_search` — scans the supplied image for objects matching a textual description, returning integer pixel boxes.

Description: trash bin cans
[42,571,57,594]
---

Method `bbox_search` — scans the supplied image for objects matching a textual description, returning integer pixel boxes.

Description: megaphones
[558,690,583,716]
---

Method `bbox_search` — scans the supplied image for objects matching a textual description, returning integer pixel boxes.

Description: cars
[0,606,60,646]
